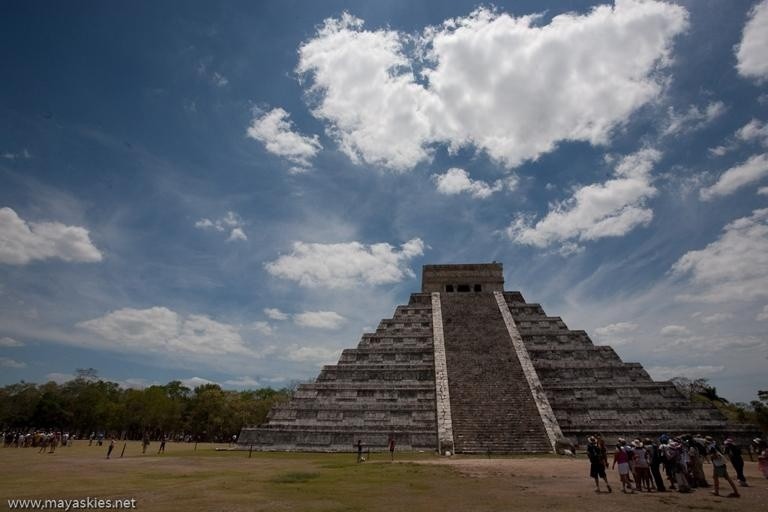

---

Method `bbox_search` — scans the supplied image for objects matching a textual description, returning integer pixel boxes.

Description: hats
[631,439,643,448]
[588,436,597,443]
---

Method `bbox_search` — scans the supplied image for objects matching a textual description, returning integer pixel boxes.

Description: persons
[585,432,768,499]
[0,429,237,459]
[357,440,361,463]
[389,439,396,461]
